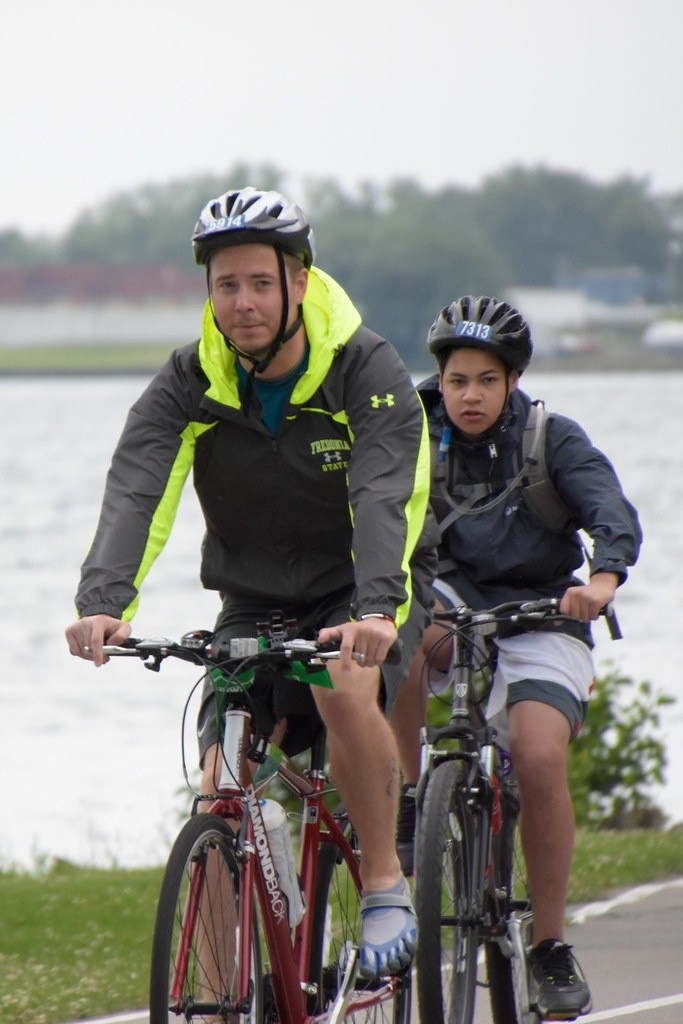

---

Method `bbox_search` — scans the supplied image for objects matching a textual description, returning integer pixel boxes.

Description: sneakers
[395,782,421,877]
[524,939,593,1022]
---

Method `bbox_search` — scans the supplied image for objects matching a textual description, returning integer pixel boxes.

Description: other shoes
[359,878,420,979]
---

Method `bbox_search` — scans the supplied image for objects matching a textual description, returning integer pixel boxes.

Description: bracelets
[359,612,397,629]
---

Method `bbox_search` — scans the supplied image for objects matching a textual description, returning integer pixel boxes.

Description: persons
[63,186,440,1024]
[384,295,644,1021]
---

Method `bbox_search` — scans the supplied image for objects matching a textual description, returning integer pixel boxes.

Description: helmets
[192,187,313,269]
[429,295,533,376]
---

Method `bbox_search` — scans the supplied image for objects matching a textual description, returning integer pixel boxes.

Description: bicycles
[78,632,416,1022]
[395,596,625,1024]
